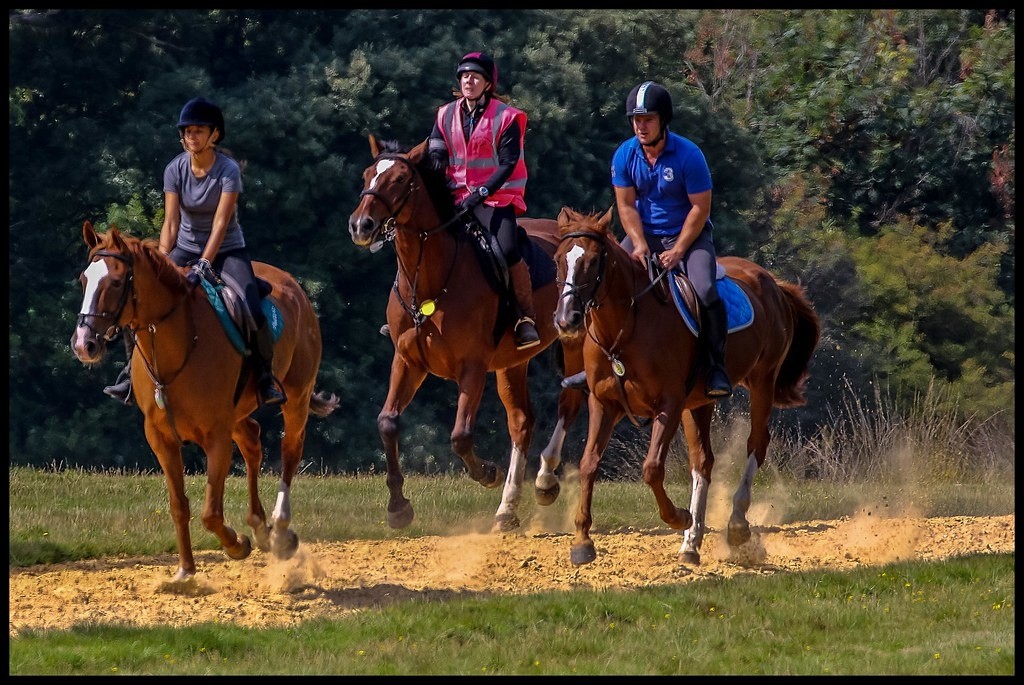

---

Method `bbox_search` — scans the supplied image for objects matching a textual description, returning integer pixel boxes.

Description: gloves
[460,189,484,210]
[432,159,445,178]
[185,261,208,288]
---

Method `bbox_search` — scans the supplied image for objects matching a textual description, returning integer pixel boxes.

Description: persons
[560,81,732,397]
[103,97,288,407]
[378,52,541,352]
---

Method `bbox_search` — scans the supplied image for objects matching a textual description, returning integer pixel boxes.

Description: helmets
[177,98,226,145]
[457,52,498,92]
[626,81,673,123]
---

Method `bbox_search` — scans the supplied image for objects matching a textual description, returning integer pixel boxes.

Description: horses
[71,134,819,585]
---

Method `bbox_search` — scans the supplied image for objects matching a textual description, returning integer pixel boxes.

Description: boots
[379,324,390,337]
[561,371,588,390]
[507,257,539,345]
[103,379,133,406]
[250,320,284,405]
[702,302,731,396]
